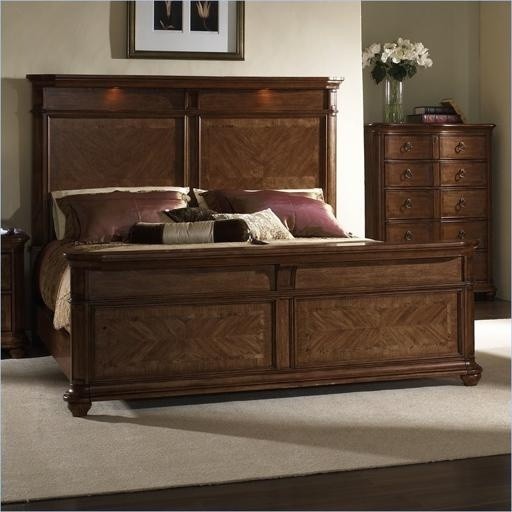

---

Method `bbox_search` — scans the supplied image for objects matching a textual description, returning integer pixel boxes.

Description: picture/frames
[128,1,246,60]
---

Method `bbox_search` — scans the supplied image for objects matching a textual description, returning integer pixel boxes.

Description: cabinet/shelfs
[366,122,499,300]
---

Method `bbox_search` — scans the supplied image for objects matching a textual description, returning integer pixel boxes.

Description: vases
[380,74,405,124]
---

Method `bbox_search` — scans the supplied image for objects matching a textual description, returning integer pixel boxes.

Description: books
[406,96,469,125]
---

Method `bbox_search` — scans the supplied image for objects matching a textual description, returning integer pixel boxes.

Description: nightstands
[1,231,31,360]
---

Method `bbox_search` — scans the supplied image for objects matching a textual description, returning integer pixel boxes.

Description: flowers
[362,37,433,124]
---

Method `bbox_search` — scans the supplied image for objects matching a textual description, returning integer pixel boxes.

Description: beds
[26,73,487,418]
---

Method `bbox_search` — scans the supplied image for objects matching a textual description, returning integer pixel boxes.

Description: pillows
[55,191,188,244]
[49,186,191,240]
[132,219,251,243]
[202,188,347,238]
[191,186,325,219]
[212,208,294,240]
[161,206,218,223]
